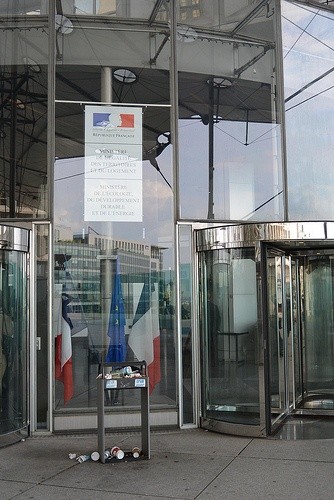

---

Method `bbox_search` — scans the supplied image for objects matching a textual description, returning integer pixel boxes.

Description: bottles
[132,447,140,457]
[104,449,110,459]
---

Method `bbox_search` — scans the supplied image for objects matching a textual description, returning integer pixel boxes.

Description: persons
[206,292,223,377]
[285,293,292,338]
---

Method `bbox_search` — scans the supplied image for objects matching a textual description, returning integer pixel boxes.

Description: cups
[91,451,99,461]
[111,446,124,459]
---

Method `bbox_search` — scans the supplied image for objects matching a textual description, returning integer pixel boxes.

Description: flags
[102,256,128,371]
[54,282,74,408]
[128,254,162,395]
